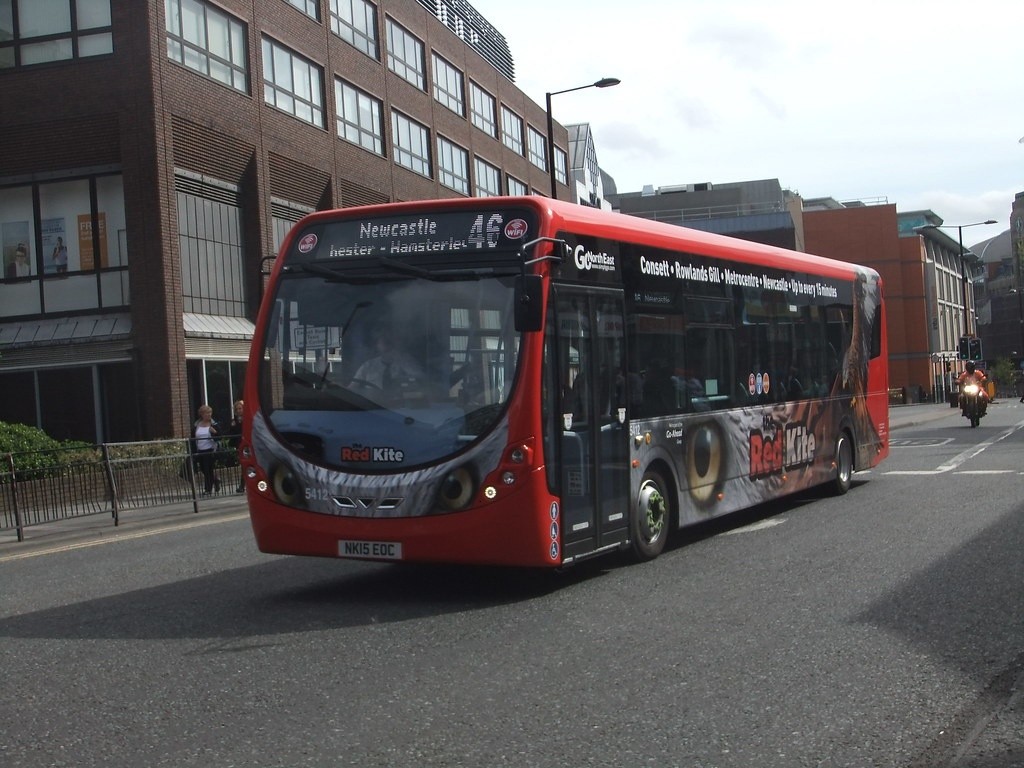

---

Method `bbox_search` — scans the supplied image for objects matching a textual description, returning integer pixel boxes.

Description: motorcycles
[953,373,991,429]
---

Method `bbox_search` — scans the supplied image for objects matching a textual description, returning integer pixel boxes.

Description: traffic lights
[971,339,983,362]
[945,362,952,373]
[958,337,969,361]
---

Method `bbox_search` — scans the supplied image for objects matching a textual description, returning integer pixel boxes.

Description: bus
[230,196,888,573]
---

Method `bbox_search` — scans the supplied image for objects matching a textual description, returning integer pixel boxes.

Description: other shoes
[202,490,212,495]
[214,478,221,491]
[1020,400,1024,403]
[236,486,245,492]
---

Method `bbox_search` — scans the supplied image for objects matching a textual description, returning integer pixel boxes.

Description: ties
[380,357,390,390]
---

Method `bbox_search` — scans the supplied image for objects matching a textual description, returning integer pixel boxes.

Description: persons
[53,237,68,273]
[230,400,248,493]
[186,406,221,495]
[954,360,989,414]
[6,247,31,284]
[346,328,424,396]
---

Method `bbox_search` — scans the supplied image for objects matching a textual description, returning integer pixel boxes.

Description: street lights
[545,75,623,199]
[922,217,999,363]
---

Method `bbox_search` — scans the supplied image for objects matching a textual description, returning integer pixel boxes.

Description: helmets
[966,360,975,374]
